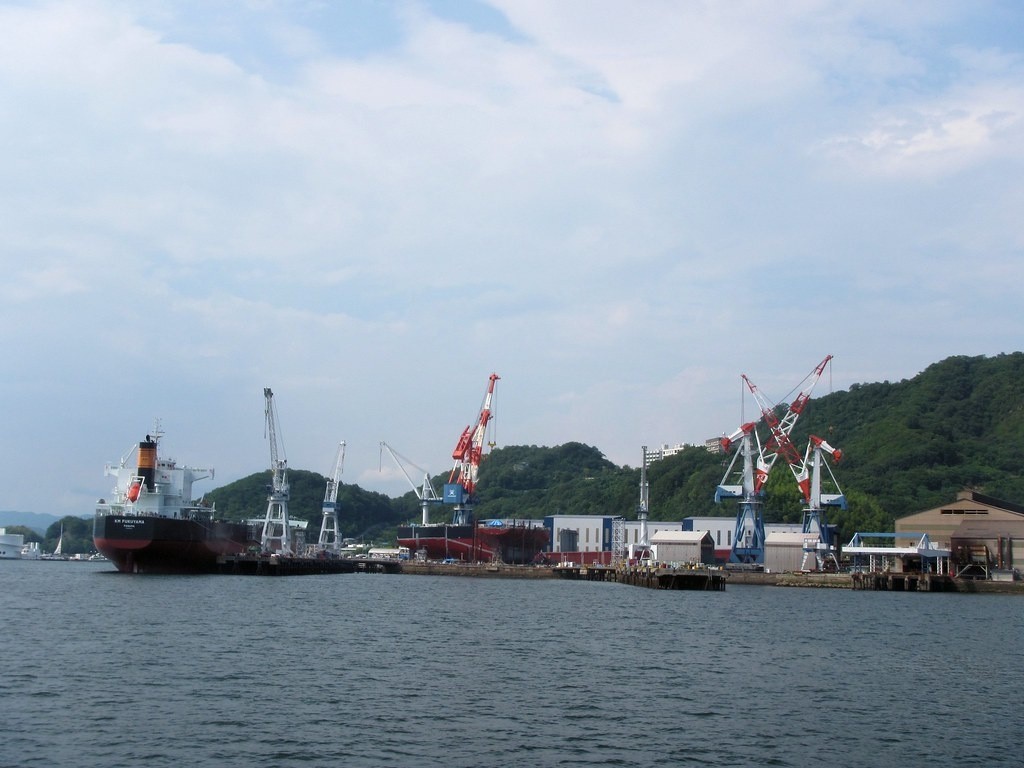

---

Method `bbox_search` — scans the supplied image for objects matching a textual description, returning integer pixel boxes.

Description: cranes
[256,373,513,558]
[631,350,857,575]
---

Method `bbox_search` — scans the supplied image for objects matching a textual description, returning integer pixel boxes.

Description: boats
[92,419,303,574]
